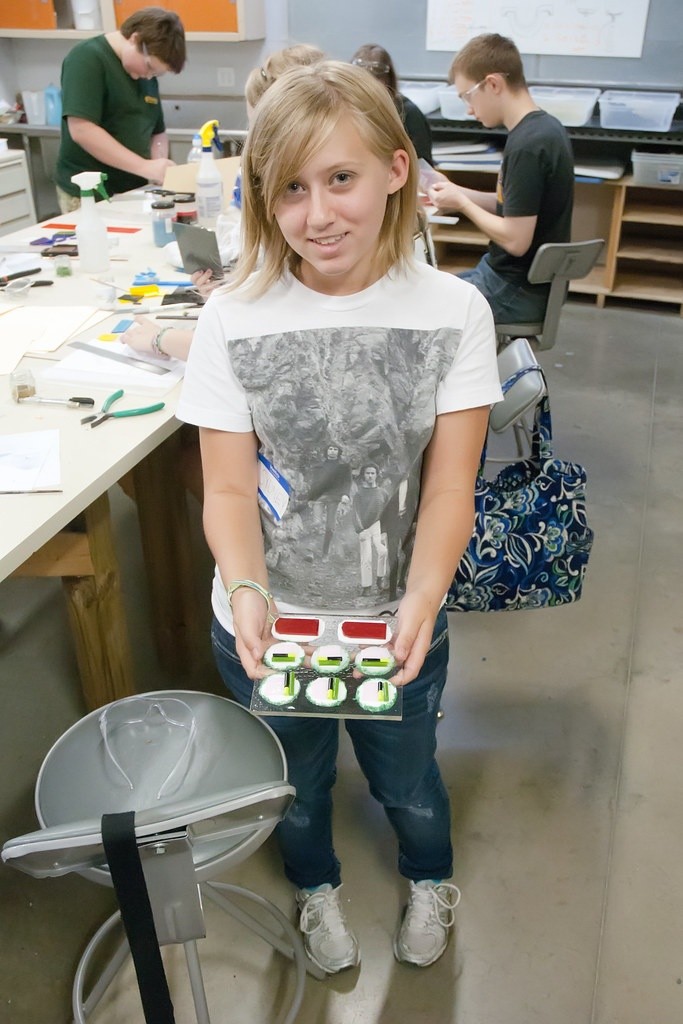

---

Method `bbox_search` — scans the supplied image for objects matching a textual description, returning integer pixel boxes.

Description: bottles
[187,133,201,163]
[152,200,197,248]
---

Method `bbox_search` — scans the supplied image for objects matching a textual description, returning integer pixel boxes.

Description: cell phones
[41,244,78,257]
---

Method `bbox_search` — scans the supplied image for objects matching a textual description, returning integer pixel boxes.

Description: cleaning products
[192,119,224,224]
[69,171,111,270]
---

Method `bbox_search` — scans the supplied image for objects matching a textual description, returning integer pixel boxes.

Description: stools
[488,233,609,466]
[1,687,307,1024]
[480,334,548,435]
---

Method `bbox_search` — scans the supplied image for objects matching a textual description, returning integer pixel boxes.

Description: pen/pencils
[133,303,198,314]
[18,397,94,409]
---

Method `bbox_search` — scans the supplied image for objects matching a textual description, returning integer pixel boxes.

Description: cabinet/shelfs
[0,0,267,44]
[420,164,683,318]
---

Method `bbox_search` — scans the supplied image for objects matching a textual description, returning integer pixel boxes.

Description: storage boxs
[630,147,683,188]
[396,79,450,116]
[597,89,681,132]
[527,85,602,128]
[439,84,478,122]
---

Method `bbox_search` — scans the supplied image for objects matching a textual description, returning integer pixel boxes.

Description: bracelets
[227,579,275,622]
[152,325,174,360]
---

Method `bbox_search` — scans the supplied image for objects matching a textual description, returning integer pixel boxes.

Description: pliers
[0,268,54,286]
[81,389,165,428]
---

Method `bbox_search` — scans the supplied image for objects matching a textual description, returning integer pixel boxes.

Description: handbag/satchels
[444,363,596,614]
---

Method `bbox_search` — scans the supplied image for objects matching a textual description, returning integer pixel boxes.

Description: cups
[0,138,8,157]
[4,277,34,297]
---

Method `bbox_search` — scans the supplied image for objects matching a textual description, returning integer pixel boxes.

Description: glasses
[140,40,166,81]
[460,72,510,108]
[351,58,391,75]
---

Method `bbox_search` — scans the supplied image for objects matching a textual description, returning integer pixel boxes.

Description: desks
[0,122,250,211]
[0,180,252,712]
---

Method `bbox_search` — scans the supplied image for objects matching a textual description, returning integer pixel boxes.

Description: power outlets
[217,68,234,88]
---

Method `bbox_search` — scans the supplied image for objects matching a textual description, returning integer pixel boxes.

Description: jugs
[21,91,46,126]
[45,81,62,126]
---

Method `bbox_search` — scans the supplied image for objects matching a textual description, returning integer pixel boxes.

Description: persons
[351,44,433,168]
[55,8,186,214]
[419,35,574,324]
[351,461,420,602]
[177,61,505,974]
[306,441,353,563]
[120,44,328,362]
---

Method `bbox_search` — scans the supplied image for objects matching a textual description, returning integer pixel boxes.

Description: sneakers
[392,880,462,968]
[295,882,362,975]
